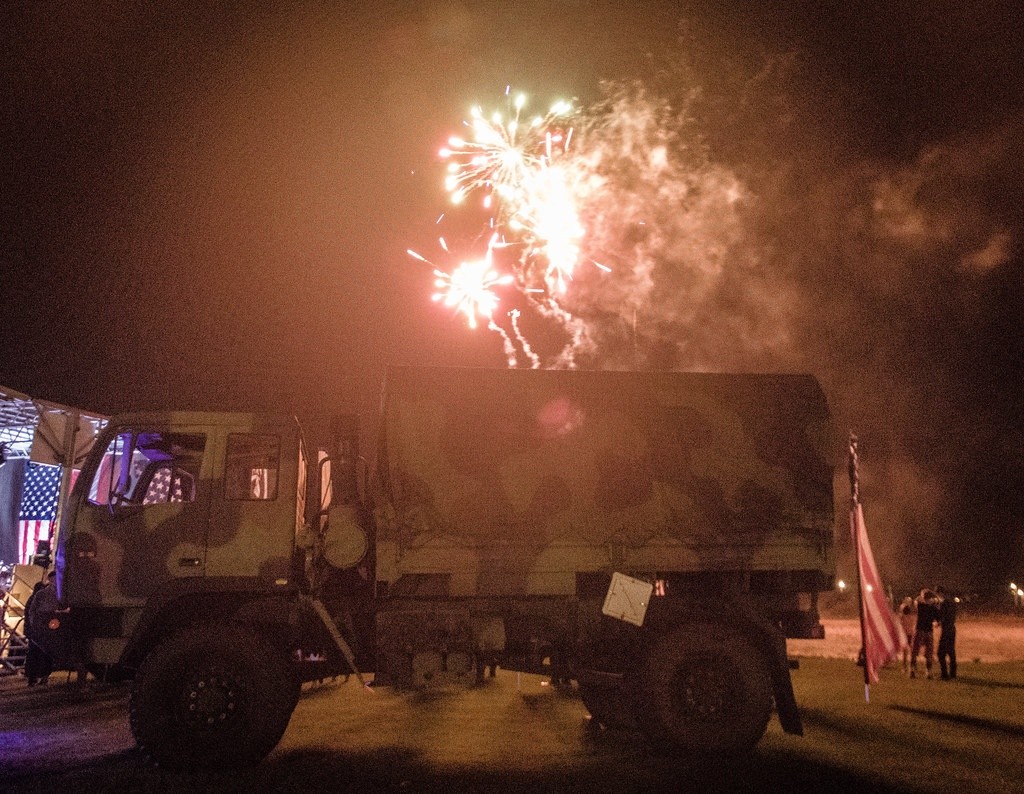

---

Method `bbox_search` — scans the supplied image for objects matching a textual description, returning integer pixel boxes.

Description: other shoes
[950,669,956,678]
[926,670,933,679]
[910,668,916,679]
[940,669,948,679]
[27,681,37,689]
[39,678,48,686]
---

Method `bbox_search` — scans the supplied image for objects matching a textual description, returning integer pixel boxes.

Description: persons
[23,572,58,690]
[898,589,958,683]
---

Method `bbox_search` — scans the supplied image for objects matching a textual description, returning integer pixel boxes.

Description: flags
[848,439,908,687]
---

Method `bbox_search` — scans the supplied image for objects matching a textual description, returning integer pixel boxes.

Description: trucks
[24,359,836,761]
